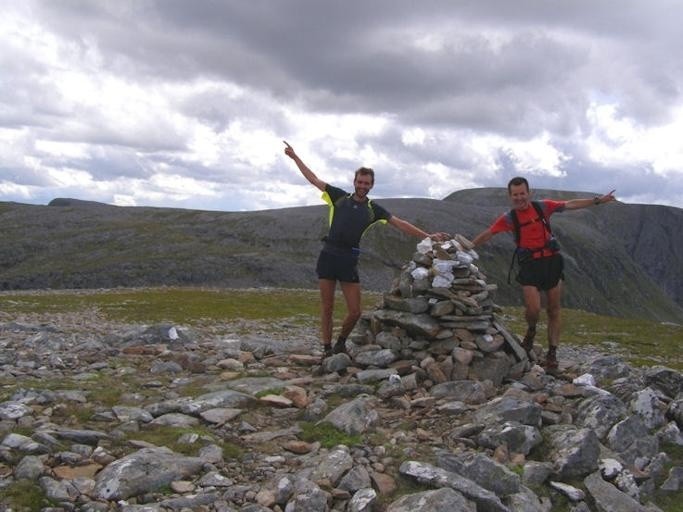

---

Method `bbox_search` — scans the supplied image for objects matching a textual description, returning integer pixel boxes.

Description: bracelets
[593,196,600,205]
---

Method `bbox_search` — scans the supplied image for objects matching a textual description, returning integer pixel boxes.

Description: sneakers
[324,346,353,359]
[523,330,535,350]
[547,350,558,367]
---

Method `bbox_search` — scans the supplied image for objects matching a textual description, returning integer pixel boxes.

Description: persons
[470,177,618,373]
[281,139,445,361]
